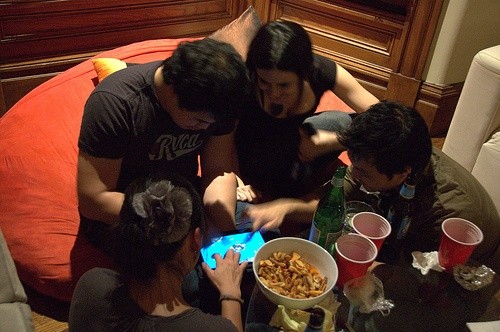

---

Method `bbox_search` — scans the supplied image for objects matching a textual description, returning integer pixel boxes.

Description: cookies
[259,252,326,299]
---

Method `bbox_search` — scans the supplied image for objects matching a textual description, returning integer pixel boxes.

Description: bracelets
[220,295,244,307]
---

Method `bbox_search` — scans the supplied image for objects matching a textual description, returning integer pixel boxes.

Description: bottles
[383,177,417,248]
[308,165,347,259]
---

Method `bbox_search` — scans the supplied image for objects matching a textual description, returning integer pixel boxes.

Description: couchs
[442,44,500,216]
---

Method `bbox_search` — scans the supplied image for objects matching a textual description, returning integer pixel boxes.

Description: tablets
[197,227,271,275]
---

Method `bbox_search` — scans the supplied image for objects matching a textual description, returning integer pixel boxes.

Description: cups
[340,200,375,234]
[352,212,392,255]
[334,233,377,290]
[438,218,483,274]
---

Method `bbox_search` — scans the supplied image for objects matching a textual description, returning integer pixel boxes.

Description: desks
[245,228,500,332]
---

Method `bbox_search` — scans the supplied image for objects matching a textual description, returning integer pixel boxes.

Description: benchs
[1,38,356,301]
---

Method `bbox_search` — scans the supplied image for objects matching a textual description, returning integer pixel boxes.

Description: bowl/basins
[252,237,338,311]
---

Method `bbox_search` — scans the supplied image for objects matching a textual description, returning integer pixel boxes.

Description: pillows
[208,5,263,61]
[91,58,143,83]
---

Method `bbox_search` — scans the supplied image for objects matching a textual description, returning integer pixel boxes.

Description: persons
[67,170,247,332]
[244,99,499,272]
[199,21,379,235]
[78,39,280,308]
